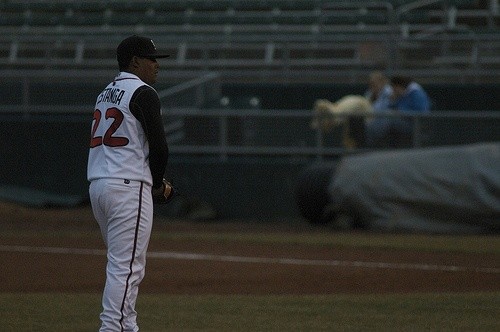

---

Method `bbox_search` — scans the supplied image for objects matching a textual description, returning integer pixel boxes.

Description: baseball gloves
[152,177,177,205]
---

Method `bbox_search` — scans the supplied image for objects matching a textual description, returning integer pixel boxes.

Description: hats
[117,35,170,69]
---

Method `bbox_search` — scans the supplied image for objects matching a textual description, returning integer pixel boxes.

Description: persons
[361,66,395,150]
[86,35,171,330]
[383,75,429,149]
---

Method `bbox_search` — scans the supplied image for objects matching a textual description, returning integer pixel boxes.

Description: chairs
[1,0,500,163]
